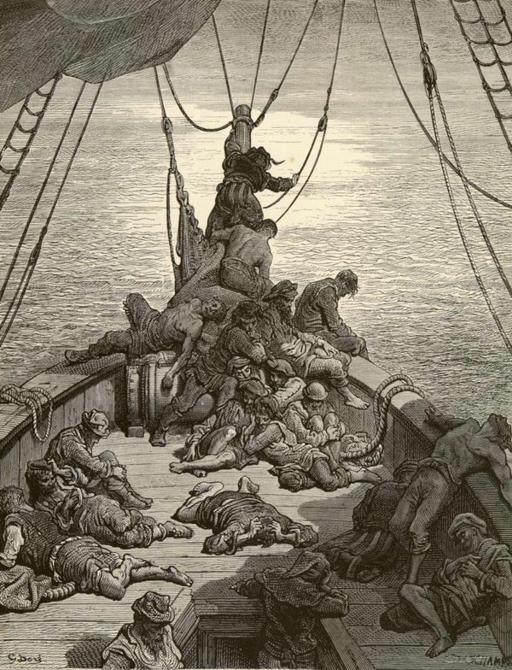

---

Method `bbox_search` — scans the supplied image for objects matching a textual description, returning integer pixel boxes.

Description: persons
[62,268,385,491]
[230,549,351,670]
[207,218,279,294]
[174,476,320,555]
[388,404,512,581]
[100,590,187,668]
[47,404,153,509]
[315,457,422,580]
[25,459,192,546]
[205,130,302,234]
[1,484,196,601]
[378,510,512,658]
